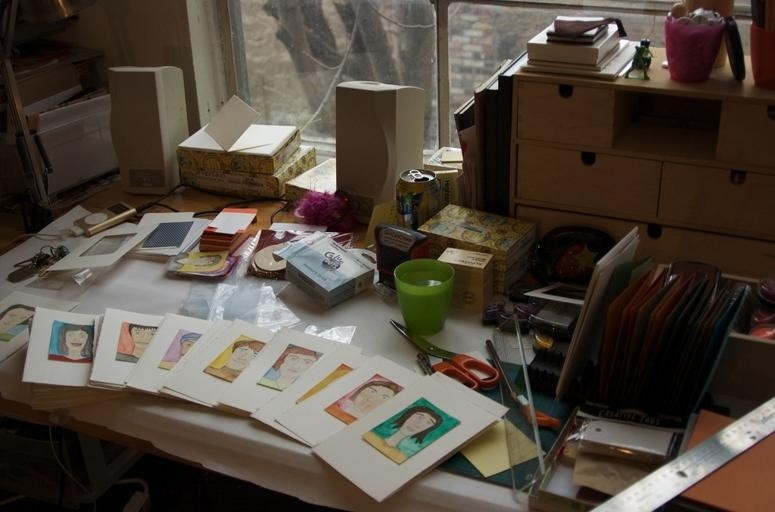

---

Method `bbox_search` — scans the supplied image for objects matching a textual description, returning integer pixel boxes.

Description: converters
[122,491,152,512]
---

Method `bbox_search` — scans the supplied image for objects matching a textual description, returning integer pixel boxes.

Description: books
[519,15,642,80]
[417,204,534,303]
[0,291,510,505]
[124,207,258,263]
[454,47,529,216]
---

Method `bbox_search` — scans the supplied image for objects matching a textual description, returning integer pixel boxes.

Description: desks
[0,177,775,511]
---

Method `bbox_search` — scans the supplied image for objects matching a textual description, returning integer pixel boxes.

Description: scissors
[390,319,500,390]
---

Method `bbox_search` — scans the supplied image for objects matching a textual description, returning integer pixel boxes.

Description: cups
[394,259,455,336]
[664,14,725,83]
[750,24,775,89]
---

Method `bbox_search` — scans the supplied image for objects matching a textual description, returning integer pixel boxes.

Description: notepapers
[199,208,259,252]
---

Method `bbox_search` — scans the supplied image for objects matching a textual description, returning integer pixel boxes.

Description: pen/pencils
[416,352,434,375]
[486,339,518,402]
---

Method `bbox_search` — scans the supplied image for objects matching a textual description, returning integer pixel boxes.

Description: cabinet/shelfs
[507,43,775,289]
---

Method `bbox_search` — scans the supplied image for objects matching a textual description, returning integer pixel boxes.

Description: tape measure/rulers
[591,397,775,512]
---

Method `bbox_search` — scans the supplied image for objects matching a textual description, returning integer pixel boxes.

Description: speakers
[109,66,189,196]
[336,81,425,224]
